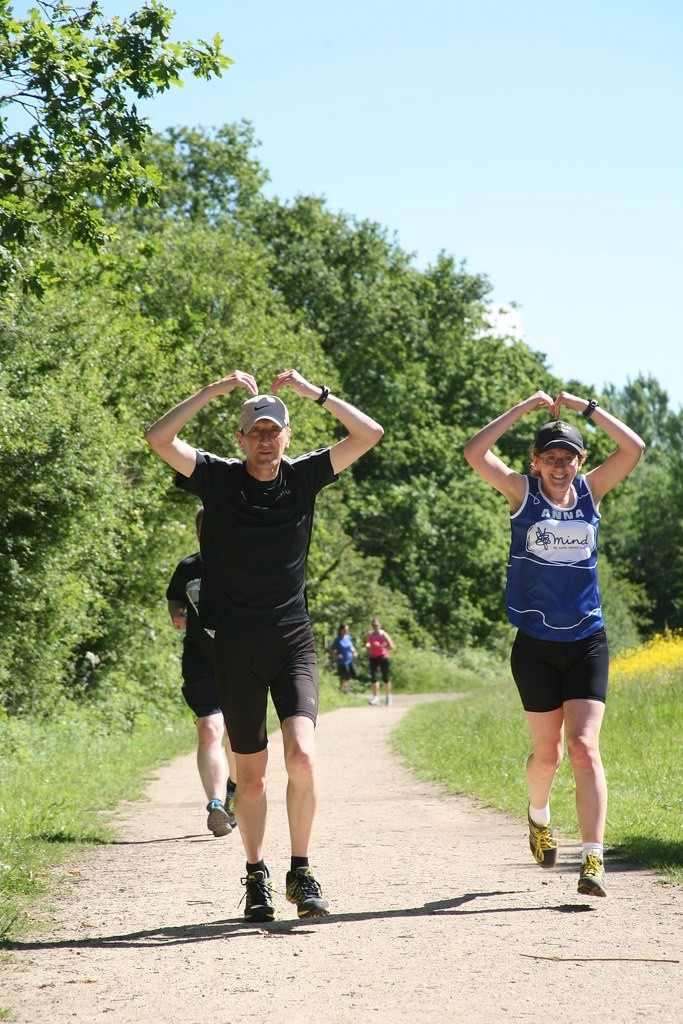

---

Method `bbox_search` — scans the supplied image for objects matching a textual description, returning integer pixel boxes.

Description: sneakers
[286,866,330,918]
[225,777,236,828]
[527,803,558,868]
[206,800,232,838]
[245,865,275,921]
[578,855,607,898]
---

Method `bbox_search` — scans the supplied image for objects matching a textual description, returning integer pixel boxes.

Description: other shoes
[385,696,391,706]
[370,698,379,705]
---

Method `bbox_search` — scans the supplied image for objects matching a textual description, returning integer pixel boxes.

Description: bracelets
[314,383,331,406]
[579,397,600,419]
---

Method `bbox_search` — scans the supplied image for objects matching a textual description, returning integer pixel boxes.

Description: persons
[332,621,360,694]
[464,388,648,898]
[364,615,396,708]
[159,504,241,840]
[142,366,388,928]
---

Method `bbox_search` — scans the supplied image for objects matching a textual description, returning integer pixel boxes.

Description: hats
[239,395,289,435]
[538,420,585,455]
